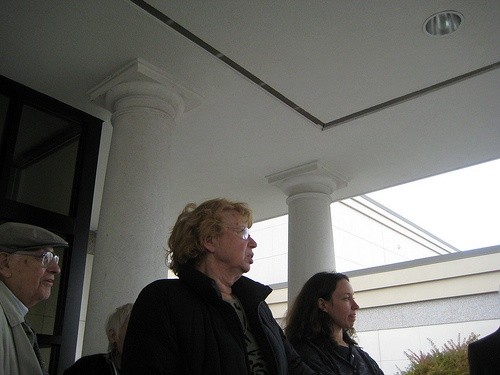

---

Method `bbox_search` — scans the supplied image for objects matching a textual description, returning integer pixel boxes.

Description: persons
[124,199,317,375]
[60,303,134,375]
[285,272,386,375]
[0,222,69,375]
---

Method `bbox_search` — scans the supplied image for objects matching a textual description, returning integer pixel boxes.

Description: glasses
[12,252,59,267]
[228,227,250,240]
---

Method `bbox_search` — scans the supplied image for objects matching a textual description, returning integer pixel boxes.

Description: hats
[0,223,69,253]
